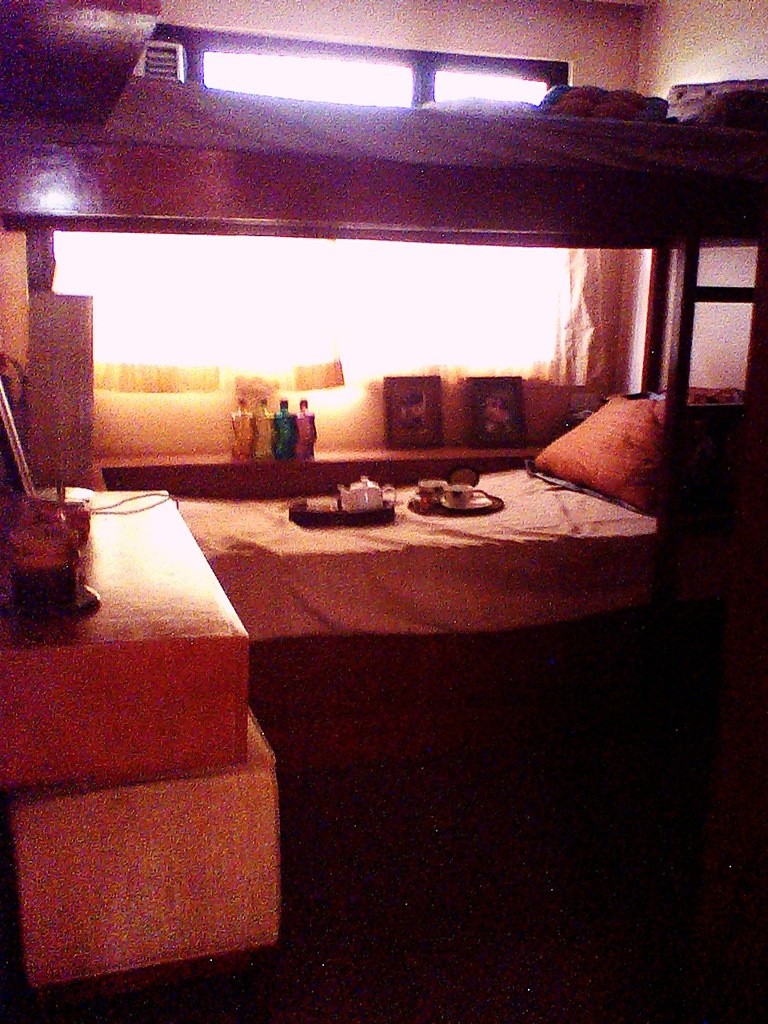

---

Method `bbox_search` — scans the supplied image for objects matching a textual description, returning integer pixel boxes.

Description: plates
[441,492,493,509]
[0,584,101,617]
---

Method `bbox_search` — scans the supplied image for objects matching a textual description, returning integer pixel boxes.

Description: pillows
[664,78,768,133]
[525,386,745,519]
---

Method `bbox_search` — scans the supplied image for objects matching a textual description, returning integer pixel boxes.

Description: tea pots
[338,476,396,512]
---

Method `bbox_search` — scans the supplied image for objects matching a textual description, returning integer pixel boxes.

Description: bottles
[230,398,255,459]
[272,399,298,460]
[251,399,275,458]
[296,400,317,457]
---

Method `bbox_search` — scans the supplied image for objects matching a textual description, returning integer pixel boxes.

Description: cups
[446,484,473,505]
[418,480,446,503]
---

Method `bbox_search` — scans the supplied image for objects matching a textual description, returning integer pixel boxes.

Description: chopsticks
[0,380,36,496]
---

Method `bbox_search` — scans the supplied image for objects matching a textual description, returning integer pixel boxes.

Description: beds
[1,78,768,247]
[171,470,733,644]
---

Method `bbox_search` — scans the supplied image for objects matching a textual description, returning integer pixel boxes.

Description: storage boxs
[1,488,252,784]
[3,704,281,992]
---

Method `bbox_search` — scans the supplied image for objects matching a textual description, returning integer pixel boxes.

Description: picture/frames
[384,377,444,450]
[463,376,525,449]
